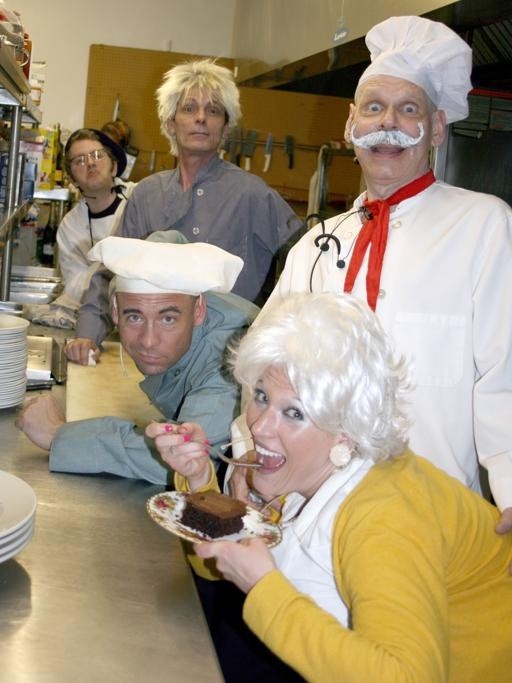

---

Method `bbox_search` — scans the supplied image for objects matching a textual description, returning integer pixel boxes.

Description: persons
[238,74,512,538]
[14,229,302,683]
[55,128,139,299]
[62,56,312,365]
[145,293,512,683]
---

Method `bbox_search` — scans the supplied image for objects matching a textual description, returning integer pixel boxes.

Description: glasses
[67,150,109,167]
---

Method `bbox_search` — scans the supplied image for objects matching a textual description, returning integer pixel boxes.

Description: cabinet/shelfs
[0,66,42,239]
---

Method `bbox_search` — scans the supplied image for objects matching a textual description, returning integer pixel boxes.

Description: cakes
[181,488,247,539]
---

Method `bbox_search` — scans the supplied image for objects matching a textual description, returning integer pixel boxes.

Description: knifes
[221,127,294,173]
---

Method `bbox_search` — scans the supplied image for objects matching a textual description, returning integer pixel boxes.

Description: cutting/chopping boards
[64,339,173,451]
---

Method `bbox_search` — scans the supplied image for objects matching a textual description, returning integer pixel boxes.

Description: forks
[154,415,261,467]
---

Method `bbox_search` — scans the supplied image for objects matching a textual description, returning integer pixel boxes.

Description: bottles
[39,200,64,268]
[52,123,65,186]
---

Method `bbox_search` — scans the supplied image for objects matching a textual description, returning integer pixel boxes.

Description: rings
[170,445,176,455]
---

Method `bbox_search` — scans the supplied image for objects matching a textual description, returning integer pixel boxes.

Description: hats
[85,236,245,298]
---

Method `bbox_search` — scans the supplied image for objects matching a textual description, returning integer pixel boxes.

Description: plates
[1,313,31,411]
[144,492,283,552]
[0,468,38,566]
